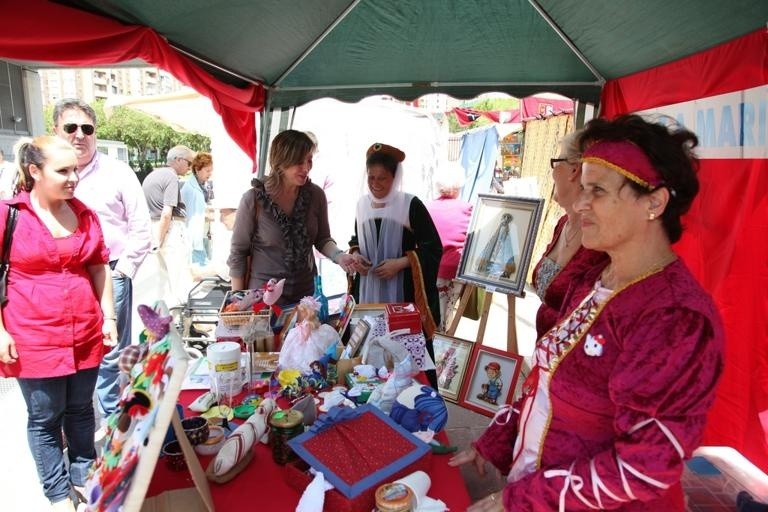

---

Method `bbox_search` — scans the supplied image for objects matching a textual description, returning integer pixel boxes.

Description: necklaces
[564,220,581,247]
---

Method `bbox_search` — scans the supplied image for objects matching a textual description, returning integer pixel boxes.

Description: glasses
[550,157,566,170]
[63,123,95,135]
[182,157,192,168]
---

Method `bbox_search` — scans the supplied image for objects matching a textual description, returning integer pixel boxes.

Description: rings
[490,493,496,501]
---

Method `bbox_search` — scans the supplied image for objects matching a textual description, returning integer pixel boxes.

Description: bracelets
[332,250,347,264]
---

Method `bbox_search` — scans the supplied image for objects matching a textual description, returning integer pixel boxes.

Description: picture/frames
[458,342,524,419]
[431,330,475,403]
[456,193,545,296]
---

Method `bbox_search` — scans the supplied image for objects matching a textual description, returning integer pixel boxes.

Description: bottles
[311,274,329,321]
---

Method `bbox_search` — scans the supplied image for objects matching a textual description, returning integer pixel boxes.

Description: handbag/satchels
[1,257,11,305]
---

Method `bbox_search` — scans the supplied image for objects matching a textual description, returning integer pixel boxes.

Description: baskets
[219,287,276,325]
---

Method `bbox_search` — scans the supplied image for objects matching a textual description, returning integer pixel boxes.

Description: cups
[178,414,211,444]
[165,438,195,472]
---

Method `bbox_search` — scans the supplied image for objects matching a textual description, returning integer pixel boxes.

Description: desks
[138,351,473,512]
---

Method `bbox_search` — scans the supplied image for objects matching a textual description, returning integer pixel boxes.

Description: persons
[0,149,16,201]
[346,143,443,392]
[180,152,213,286]
[53,97,153,441]
[141,145,198,315]
[227,129,358,308]
[447,112,724,512]
[536,118,614,346]
[0,134,120,512]
[421,161,473,332]
[278,295,344,376]
